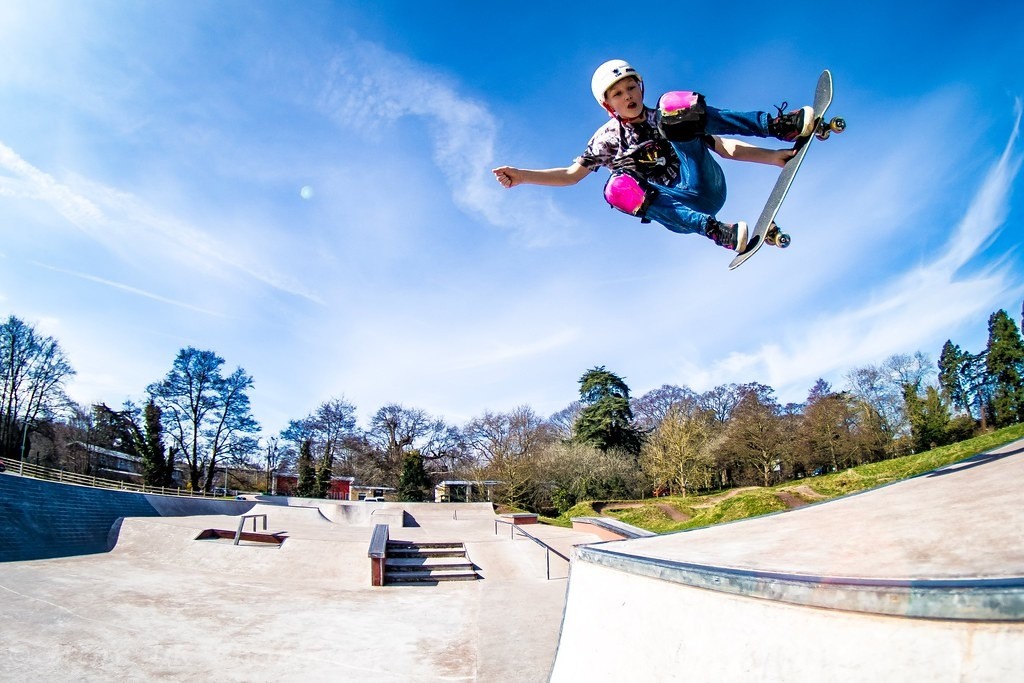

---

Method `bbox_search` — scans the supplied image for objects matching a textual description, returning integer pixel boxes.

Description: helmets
[592,60,642,111]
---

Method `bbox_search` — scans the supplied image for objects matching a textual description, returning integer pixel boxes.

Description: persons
[491,59,815,254]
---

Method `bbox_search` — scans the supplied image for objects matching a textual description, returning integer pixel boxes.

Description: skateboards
[726,68,847,271]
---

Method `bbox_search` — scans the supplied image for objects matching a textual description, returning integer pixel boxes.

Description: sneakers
[768,102,814,142]
[705,216,747,253]
